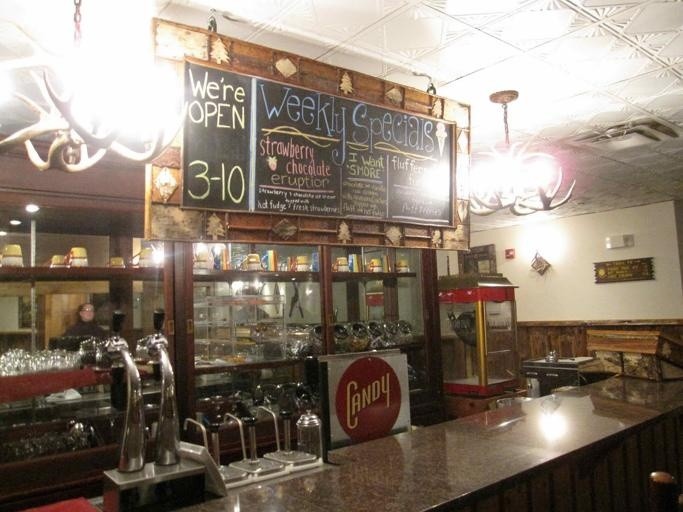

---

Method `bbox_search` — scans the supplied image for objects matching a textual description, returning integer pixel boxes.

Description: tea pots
[544,350,557,364]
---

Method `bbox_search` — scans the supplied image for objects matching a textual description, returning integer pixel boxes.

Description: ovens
[524,368,579,397]
[521,354,597,367]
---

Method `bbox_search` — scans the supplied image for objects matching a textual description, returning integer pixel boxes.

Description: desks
[532,356,594,387]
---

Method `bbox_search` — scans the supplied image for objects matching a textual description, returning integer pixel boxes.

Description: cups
[0,243,160,271]
[192,243,412,276]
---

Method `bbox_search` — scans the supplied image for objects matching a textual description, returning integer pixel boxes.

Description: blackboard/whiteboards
[180,58,458,230]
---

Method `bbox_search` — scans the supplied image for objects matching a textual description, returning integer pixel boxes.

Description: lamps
[465,90,578,217]
[0,0,190,173]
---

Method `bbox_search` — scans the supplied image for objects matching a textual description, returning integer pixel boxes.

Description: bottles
[297,412,321,462]
[295,466,321,498]
[0,321,419,463]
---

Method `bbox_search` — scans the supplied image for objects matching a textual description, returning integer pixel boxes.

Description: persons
[234,287,270,323]
[64,303,105,339]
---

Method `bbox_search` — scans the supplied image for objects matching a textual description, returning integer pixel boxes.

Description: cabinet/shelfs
[2,168,146,489]
[173,234,444,430]
